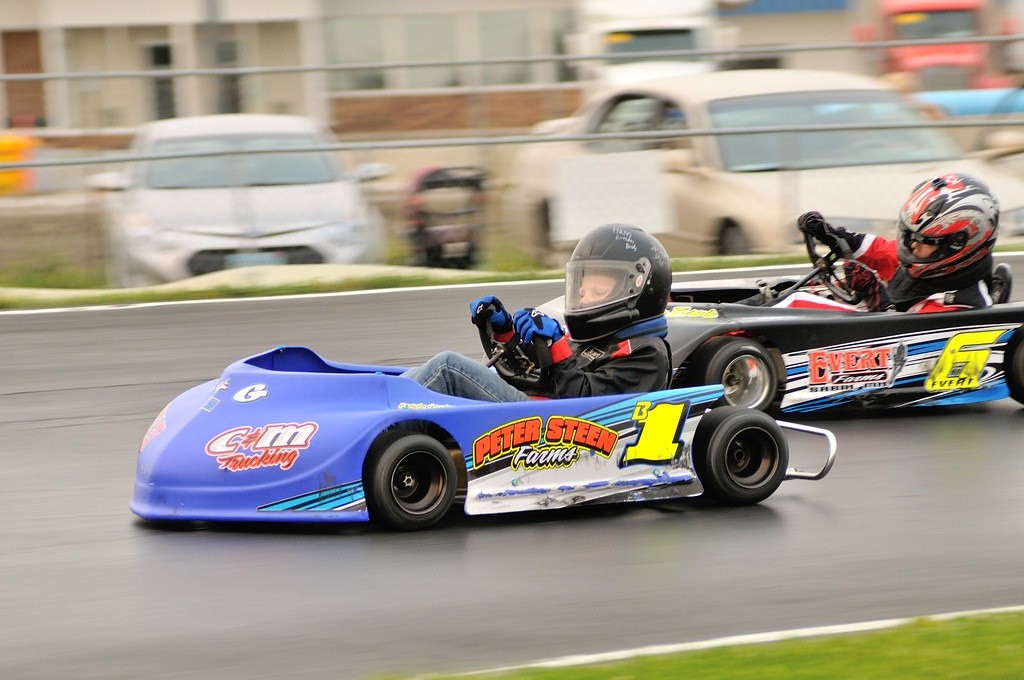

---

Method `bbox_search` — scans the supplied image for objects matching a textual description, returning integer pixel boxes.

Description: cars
[510,63,1024,255]
[85,114,390,287]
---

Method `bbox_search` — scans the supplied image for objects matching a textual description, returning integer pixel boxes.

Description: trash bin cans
[409,166,488,268]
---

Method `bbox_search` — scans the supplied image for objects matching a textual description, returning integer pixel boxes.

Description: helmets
[564,222,673,343]
[896,173,1001,280]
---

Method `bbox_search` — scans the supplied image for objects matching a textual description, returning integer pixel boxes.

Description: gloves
[512,307,573,363]
[843,260,894,312]
[797,210,846,249]
[469,295,515,343]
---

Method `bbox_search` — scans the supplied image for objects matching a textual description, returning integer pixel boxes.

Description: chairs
[993,262,1012,304]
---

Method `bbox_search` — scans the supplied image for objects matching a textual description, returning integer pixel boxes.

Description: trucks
[558,0,745,86]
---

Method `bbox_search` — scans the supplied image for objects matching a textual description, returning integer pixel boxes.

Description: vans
[852,0,1016,96]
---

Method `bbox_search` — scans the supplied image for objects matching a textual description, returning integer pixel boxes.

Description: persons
[734,171,999,312]
[397,224,677,401]
